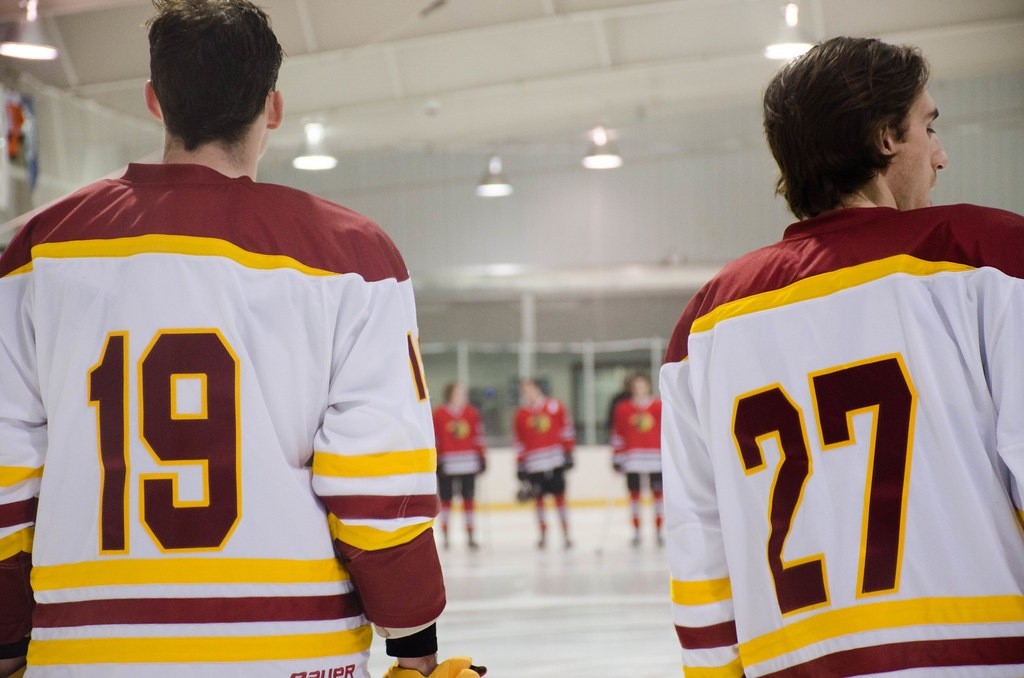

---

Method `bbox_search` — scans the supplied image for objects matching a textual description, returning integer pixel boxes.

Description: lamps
[764,1,821,60]
[293,121,337,170]
[0,0,58,60]
[475,156,512,198]
[584,126,623,170]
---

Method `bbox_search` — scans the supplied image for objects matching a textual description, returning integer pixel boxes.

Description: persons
[515,378,575,549]
[659,35,1024,678]
[611,377,667,543]
[433,384,487,546]
[0,0,487,678]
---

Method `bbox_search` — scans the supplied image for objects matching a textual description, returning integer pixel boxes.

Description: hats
[530,370,554,399]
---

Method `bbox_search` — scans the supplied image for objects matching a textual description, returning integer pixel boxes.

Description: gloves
[517,470,527,480]
[562,452,574,470]
[382,655,487,678]
[480,456,488,473]
[613,462,623,471]
[437,463,446,479]
